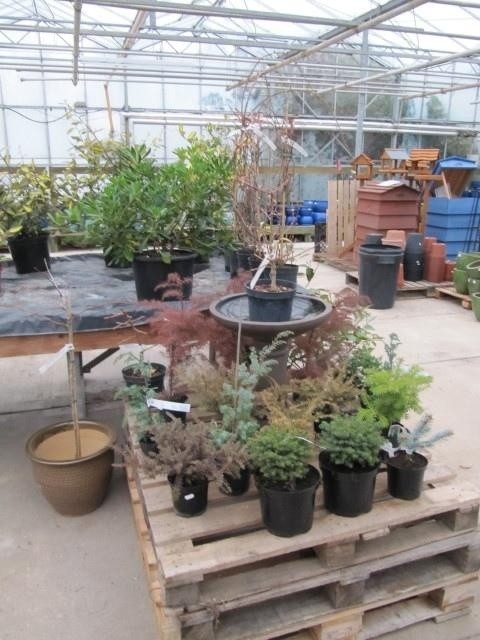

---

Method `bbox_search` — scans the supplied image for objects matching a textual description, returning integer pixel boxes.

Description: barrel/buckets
[358,244,403,310]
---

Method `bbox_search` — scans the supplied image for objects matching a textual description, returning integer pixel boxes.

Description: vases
[26,419,118,515]
[271,200,327,225]
[358,229,480,320]
[223,243,301,320]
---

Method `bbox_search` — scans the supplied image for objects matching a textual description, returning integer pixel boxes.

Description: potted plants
[93,142,231,302]
[0,158,54,274]
[109,310,432,539]
